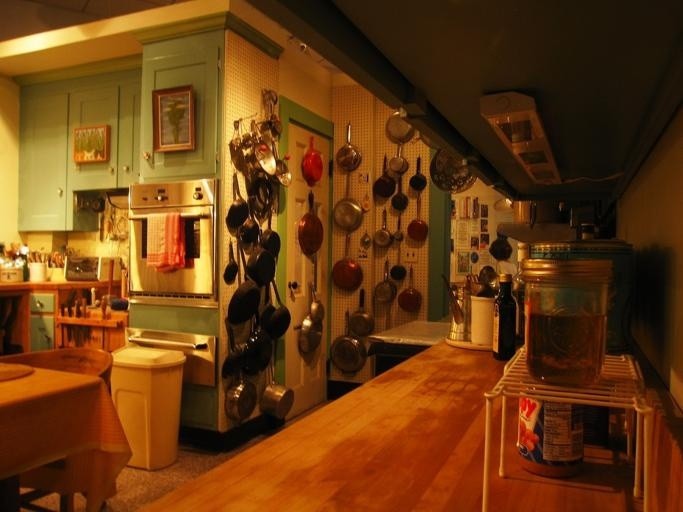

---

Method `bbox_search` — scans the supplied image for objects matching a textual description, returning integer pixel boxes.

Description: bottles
[520,259,612,387]
[517,381,585,478]
[493,273,520,361]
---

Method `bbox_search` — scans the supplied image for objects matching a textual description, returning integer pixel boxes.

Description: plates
[444,336,493,353]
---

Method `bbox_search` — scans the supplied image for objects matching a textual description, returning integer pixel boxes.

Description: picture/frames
[72,123,110,163]
[152,84,198,154]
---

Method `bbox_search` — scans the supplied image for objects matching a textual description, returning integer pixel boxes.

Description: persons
[167,99,183,143]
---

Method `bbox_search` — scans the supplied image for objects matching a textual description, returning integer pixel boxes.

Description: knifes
[58,288,96,351]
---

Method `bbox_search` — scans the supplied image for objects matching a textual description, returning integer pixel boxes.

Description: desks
[0,361,103,512]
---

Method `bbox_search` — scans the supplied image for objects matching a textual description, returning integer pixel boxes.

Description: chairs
[0,347,113,397]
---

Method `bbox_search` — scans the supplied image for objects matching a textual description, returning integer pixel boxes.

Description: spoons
[393,212,403,241]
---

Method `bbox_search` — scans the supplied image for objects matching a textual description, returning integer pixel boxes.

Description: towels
[156,217,186,272]
[147,212,180,265]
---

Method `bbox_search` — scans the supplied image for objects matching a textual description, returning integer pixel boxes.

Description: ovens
[126,177,219,300]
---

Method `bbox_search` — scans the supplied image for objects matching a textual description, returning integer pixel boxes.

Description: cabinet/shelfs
[134,341,628,512]
[19,76,98,231]
[69,68,141,189]
[0,281,122,353]
[369,319,453,377]
[484,346,647,512]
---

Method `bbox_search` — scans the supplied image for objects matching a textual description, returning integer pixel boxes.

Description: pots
[407,192,428,242]
[390,175,408,211]
[332,174,362,233]
[336,123,361,174]
[385,111,415,157]
[239,196,259,244]
[374,208,392,248]
[301,136,323,187]
[246,229,275,286]
[257,284,276,323]
[227,275,261,326]
[372,154,396,198]
[224,373,257,424]
[390,240,406,281]
[225,173,248,228]
[330,311,367,376]
[258,211,280,260]
[229,120,291,187]
[297,190,323,257]
[221,317,247,379]
[397,267,422,314]
[349,288,374,337]
[331,234,363,293]
[222,239,238,286]
[374,260,396,306]
[409,157,426,191]
[261,367,295,420]
[389,142,408,174]
[267,280,291,342]
[310,290,324,322]
[298,314,322,356]
[247,311,272,374]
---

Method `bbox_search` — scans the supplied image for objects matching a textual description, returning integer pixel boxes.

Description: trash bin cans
[110,342,186,470]
